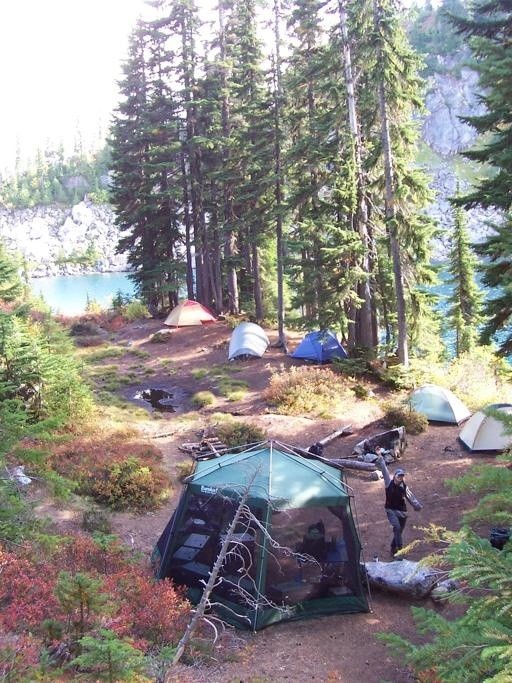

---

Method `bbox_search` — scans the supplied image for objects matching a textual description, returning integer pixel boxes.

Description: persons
[374,444,423,559]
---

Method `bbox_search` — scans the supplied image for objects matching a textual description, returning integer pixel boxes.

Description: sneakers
[390,547,402,558]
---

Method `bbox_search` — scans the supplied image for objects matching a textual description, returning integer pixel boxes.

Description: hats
[395,469,405,478]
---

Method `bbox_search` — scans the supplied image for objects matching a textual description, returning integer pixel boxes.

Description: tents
[149,444,370,632]
[162,299,219,325]
[289,329,348,361]
[226,321,272,360]
[407,383,473,426]
[458,402,512,450]
